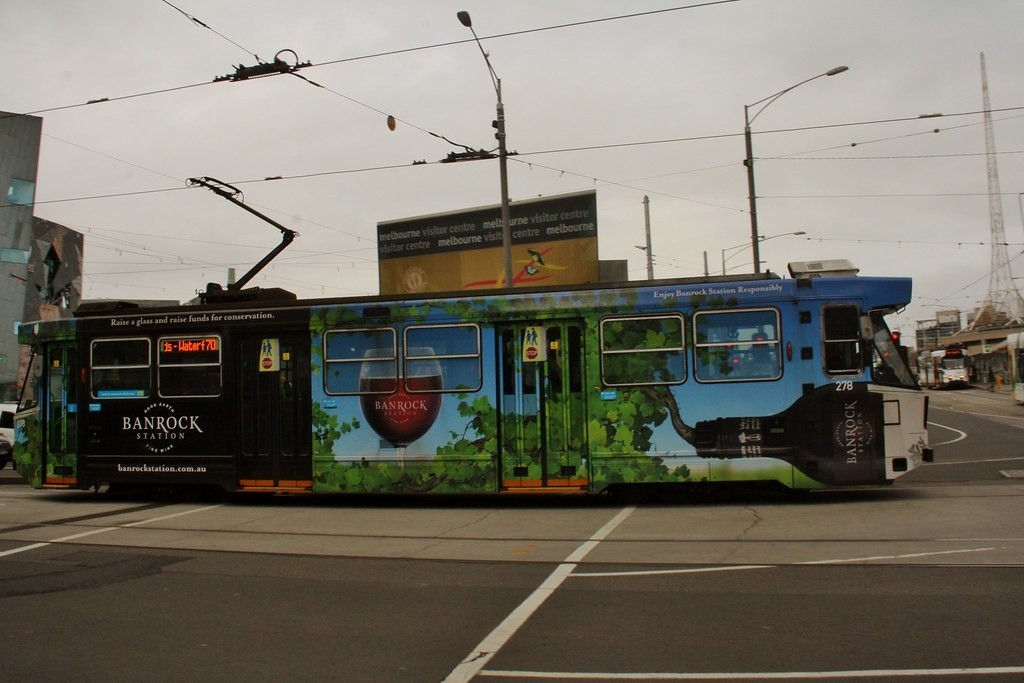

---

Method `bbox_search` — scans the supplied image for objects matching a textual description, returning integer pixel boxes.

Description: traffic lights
[892,332,901,346]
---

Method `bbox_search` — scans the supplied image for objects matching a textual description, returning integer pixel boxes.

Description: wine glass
[360,347,443,479]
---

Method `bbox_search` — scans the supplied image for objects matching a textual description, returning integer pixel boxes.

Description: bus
[917,348,970,390]
[14,276,936,498]
[1007,332,1024,402]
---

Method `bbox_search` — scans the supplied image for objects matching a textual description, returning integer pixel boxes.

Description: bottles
[695,381,897,486]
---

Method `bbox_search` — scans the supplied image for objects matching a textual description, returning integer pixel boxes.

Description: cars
[0,404,24,469]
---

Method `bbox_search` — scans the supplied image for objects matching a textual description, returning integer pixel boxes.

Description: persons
[987,364,995,392]
[968,364,979,384]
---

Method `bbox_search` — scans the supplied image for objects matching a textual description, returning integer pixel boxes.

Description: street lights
[722,230,806,276]
[743,66,849,279]
[456,10,511,289]
